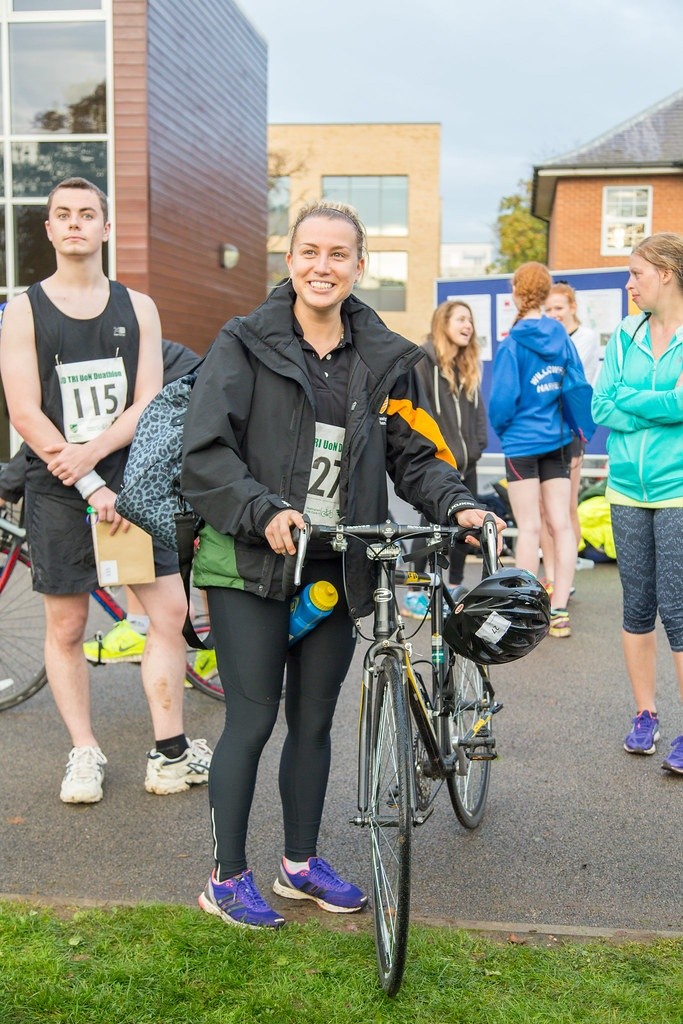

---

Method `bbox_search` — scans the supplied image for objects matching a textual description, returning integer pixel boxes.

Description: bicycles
[285,512,514,996]
[0,463,229,720]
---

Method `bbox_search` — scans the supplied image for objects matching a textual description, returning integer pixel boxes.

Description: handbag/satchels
[113,354,218,551]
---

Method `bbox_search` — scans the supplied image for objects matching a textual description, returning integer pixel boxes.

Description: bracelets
[73,468,107,499]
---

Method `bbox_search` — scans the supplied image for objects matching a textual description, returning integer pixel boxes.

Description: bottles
[288,581,338,646]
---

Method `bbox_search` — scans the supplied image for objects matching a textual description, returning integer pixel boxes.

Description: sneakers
[59,746,108,804]
[623,709,660,754]
[661,735,683,773]
[272,855,368,913]
[82,619,146,664]
[144,736,213,795]
[198,867,286,931]
[184,647,219,688]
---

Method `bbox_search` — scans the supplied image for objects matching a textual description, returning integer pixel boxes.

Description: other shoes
[575,557,595,570]
[399,590,431,620]
[539,575,576,600]
[548,608,572,637]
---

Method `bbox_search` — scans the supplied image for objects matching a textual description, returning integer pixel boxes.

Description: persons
[0,336,219,688]
[401,301,487,618]
[591,232,683,774]
[489,261,583,637]
[179,201,507,928]
[1,175,214,804]
[539,278,595,598]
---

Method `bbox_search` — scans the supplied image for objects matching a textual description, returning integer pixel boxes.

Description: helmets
[441,566,551,666]
